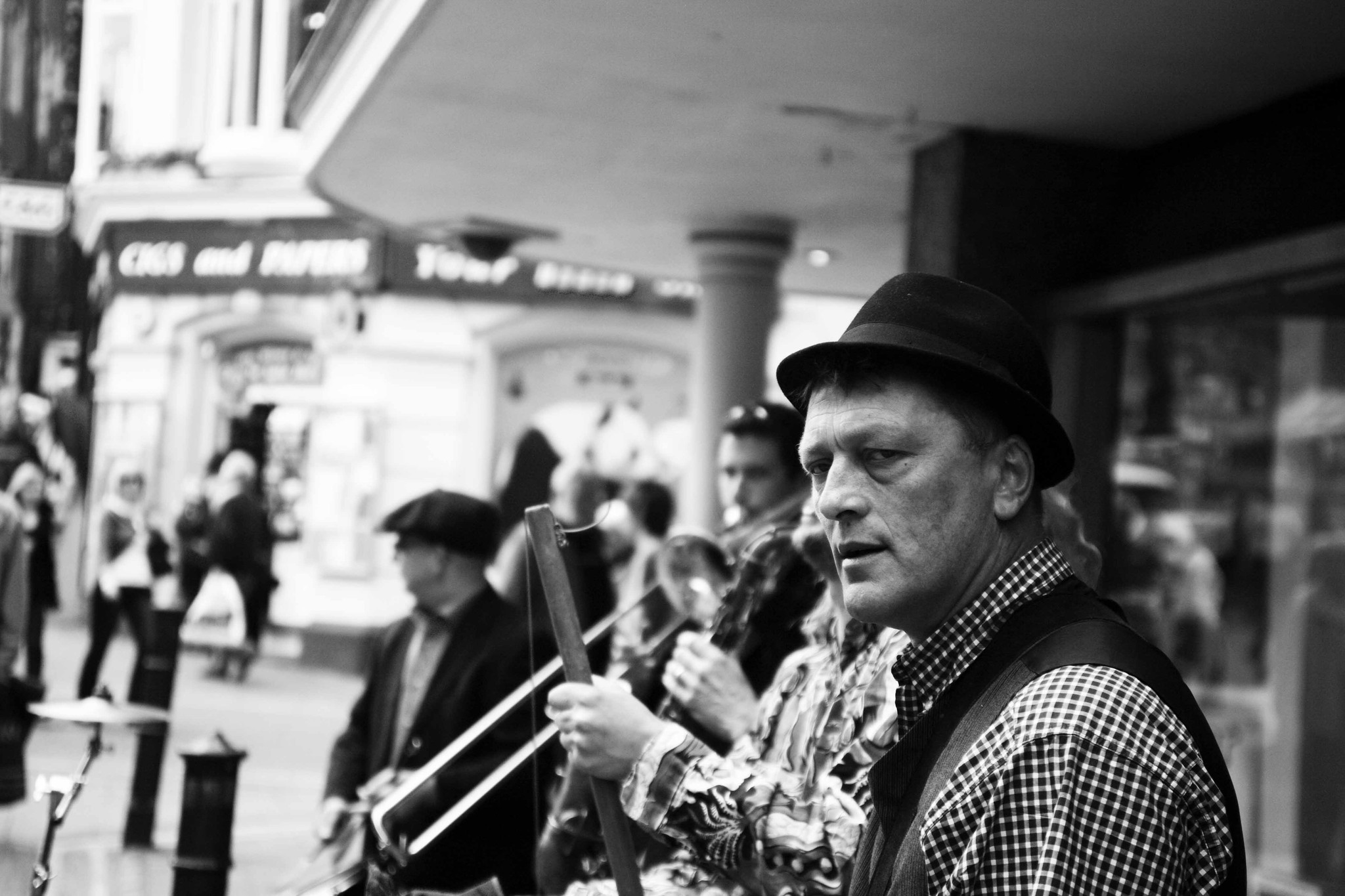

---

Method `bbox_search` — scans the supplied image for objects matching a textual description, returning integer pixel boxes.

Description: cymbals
[23,697,173,727]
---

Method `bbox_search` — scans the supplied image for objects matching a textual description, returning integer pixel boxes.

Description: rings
[675,667,686,682]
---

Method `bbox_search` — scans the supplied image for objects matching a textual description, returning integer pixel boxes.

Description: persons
[75,450,271,698]
[0,308,76,806]
[777,270,1248,895]
[314,398,920,896]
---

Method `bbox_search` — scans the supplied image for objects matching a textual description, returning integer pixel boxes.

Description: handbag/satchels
[177,570,245,649]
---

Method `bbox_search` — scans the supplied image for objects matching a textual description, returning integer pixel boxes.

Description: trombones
[369,486,815,864]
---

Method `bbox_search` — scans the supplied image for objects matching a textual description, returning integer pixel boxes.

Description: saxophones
[656,525,793,730]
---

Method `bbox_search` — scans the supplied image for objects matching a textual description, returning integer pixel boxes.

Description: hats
[376,489,501,562]
[776,272,1075,491]
